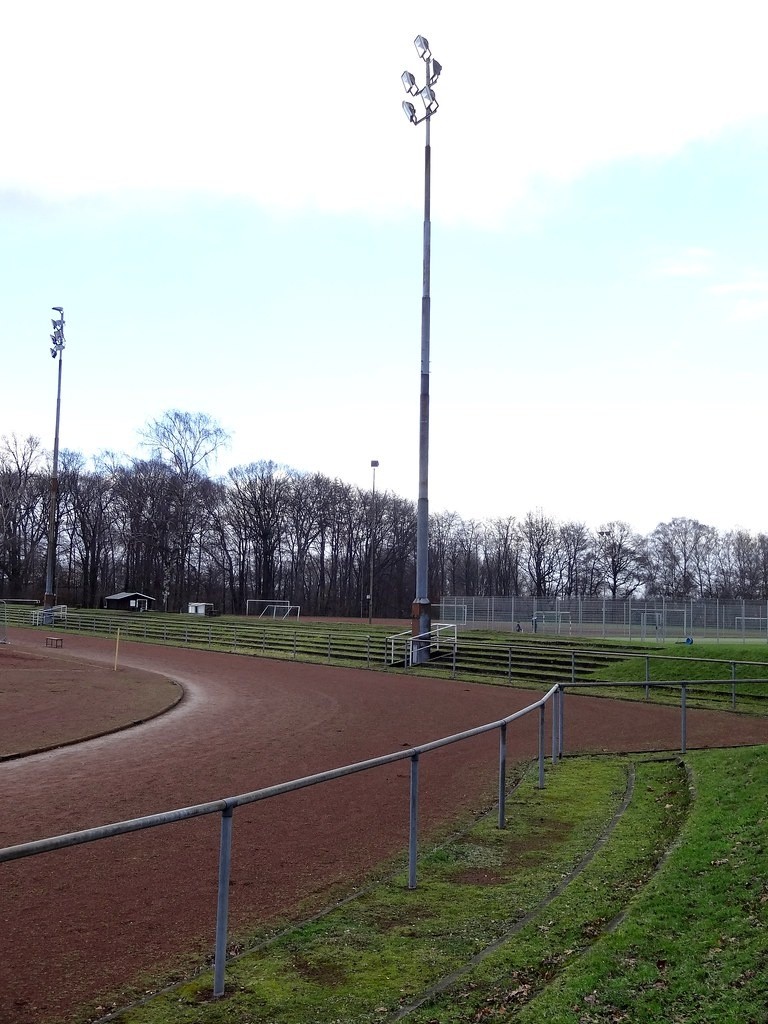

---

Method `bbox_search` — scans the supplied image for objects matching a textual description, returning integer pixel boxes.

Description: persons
[514,624,522,632]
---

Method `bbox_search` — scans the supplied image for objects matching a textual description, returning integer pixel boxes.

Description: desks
[45,636,64,649]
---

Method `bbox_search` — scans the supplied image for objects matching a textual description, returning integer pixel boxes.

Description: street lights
[398,32,443,665]
[598,531,611,636]
[42,306,67,626]
[368,458,380,624]
